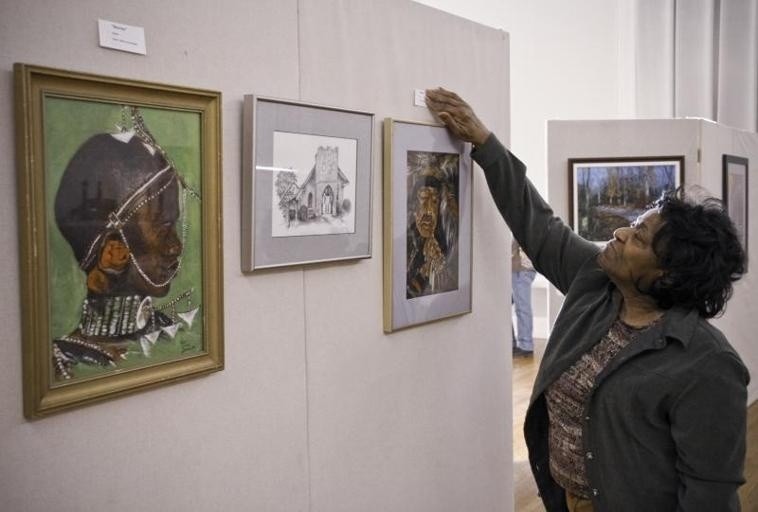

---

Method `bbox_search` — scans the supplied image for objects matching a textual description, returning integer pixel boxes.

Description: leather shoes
[512,349,532,357]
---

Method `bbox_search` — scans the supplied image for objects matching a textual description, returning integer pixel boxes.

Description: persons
[407,150,459,298]
[512,232,538,357]
[424,86,752,512]
[49,131,203,382]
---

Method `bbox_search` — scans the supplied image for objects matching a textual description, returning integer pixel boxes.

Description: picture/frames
[722,154,747,273]
[14,62,224,420]
[384,118,471,334]
[568,157,684,248]
[243,95,373,272]
